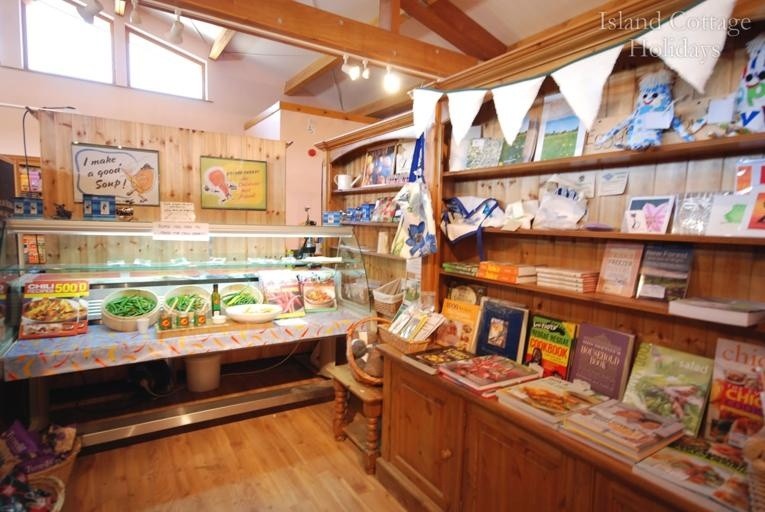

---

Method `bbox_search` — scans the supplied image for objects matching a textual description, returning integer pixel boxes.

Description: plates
[0,216,369,443]
[312,112,421,322]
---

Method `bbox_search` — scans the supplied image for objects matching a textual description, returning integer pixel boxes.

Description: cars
[136,318,149,335]
[157,310,206,331]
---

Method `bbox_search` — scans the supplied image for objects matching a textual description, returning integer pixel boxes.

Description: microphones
[329,362,383,475]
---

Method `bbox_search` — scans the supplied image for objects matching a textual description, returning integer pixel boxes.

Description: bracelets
[303,287,335,309]
[211,314,226,325]
[226,302,282,324]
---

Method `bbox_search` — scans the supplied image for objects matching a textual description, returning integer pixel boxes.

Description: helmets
[19,298,77,324]
[75,298,87,320]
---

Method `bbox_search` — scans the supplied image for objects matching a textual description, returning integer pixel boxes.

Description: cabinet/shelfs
[210,283,220,318]
[312,112,421,322]
[372,0,765,511]
[0,216,369,443]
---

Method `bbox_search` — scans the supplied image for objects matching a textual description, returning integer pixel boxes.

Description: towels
[183,354,221,392]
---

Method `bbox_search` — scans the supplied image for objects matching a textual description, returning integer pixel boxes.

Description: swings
[297,220,317,262]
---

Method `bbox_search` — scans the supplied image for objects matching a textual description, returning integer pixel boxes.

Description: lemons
[333,172,361,191]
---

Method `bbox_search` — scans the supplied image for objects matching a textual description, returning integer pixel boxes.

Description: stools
[329,362,383,475]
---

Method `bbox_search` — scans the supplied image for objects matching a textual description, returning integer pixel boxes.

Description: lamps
[74,0,104,25]
[341,53,361,82]
[166,6,186,47]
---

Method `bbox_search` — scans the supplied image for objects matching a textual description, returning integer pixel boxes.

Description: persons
[352,115,765,510]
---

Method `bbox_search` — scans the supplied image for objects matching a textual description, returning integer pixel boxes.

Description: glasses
[341,53,361,82]
[74,0,104,25]
[166,6,186,47]
